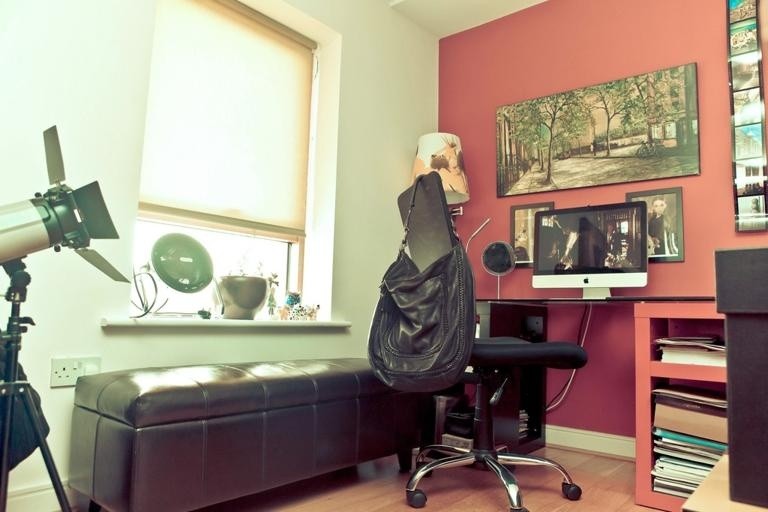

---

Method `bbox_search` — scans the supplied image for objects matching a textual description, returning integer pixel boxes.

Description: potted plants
[218,260,278,319]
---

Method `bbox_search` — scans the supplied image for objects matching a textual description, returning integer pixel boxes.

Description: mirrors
[481,241,516,300]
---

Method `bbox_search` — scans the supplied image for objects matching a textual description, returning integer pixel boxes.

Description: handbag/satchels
[368,238,476,393]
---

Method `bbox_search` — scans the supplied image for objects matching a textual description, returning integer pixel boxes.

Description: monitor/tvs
[531,200,649,299]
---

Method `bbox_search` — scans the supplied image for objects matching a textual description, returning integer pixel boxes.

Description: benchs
[68,358,421,512]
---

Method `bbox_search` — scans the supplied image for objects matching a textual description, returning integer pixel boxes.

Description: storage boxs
[716,248,767,508]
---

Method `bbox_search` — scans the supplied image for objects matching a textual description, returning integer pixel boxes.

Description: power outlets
[50,359,78,387]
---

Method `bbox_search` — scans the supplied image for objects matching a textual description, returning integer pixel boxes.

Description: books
[651,334,728,500]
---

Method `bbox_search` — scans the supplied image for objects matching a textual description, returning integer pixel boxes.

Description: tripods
[0,302,79,512]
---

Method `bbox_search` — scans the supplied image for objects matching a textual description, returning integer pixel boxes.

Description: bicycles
[637,138,667,157]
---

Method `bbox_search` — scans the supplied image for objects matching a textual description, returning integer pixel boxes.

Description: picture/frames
[510,202,554,268]
[625,188,685,262]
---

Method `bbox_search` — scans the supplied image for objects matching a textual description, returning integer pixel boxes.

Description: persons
[605,223,622,256]
[648,196,678,255]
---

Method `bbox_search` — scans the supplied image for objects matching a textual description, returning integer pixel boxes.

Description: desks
[422,298,729,512]
[683,451,767,512]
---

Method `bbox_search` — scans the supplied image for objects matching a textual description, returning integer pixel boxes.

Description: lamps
[411,132,470,205]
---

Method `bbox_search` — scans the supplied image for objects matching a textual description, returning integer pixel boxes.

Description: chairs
[398,172,588,512]
